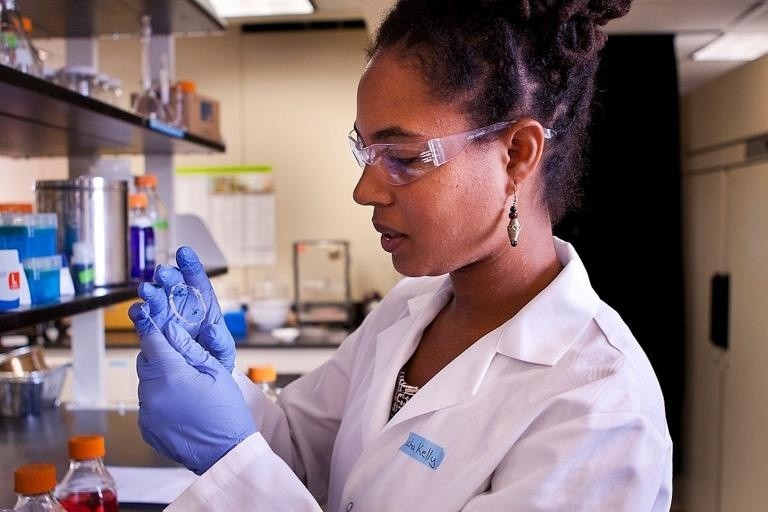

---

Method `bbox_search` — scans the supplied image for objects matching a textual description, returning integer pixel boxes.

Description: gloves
[129,244,260,478]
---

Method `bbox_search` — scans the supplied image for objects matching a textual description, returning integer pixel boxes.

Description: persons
[128,0,675,512]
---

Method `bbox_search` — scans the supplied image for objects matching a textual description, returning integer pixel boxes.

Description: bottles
[9,434,121,510]
[1,13,194,136]
[69,242,96,292]
[131,174,170,280]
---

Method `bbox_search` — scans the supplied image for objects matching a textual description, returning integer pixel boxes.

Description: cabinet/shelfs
[1,62,228,330]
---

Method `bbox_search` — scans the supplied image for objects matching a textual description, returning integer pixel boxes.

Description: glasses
[347,120,558,187]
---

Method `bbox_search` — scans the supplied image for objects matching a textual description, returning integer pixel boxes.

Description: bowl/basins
[253,301,299,344]
